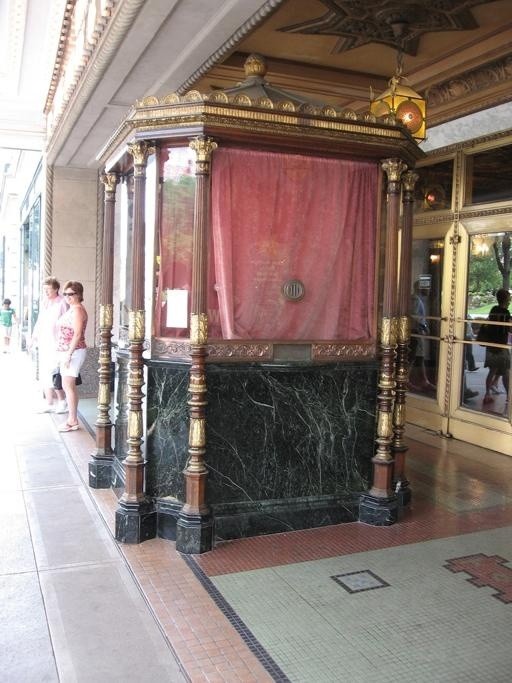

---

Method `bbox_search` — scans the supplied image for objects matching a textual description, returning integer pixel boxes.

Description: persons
[463,313,478,399]
[27,276,88,432]
[0,299,20,352]
[409,279,440,390]
[479,288,512,404]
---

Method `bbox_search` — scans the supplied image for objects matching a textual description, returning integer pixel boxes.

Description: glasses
[63,293,77,297]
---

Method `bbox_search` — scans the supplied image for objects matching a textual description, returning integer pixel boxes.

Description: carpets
[182,502,511,683]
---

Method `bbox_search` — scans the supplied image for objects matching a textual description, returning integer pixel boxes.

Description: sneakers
[45,402,67,414]
[59,422,79,431]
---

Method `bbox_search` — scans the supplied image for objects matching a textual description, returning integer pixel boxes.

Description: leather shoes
[464,366,508,404]
[409,380,436,393]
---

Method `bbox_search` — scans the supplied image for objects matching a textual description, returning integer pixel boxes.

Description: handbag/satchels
[53,373,82,390]
[476,322,509,349]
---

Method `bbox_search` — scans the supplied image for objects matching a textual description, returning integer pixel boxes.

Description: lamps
[370,19,429,147]
